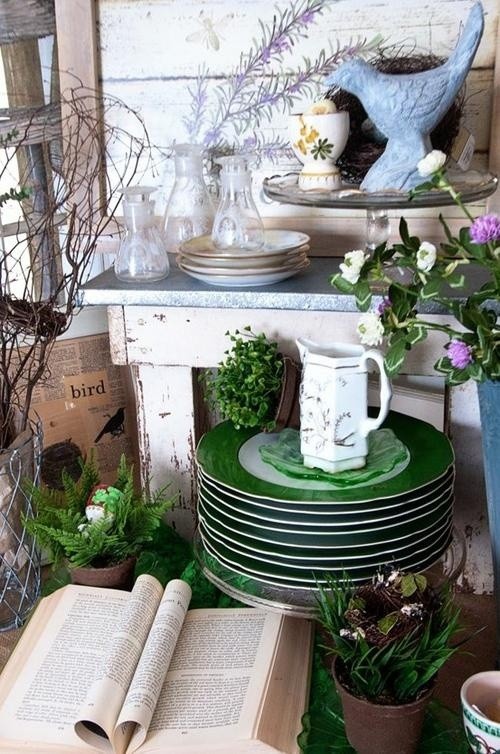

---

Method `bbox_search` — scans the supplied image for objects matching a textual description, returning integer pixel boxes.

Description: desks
[82,251,500,543]
[264,167,497,253]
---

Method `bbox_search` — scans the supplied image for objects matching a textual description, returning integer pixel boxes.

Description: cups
[460,670,500,754]
[295,334,392,475]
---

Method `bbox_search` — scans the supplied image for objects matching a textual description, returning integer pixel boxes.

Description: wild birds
[320,1,488,197]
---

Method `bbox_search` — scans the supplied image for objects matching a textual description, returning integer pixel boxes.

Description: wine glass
[284,112,350,192]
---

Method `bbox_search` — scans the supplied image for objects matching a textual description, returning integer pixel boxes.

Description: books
[0,574,317,754]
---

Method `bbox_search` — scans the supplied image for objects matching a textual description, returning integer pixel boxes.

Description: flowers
[329,147,499,386]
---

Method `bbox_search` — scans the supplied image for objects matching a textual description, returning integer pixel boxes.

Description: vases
[1,431,36,590]
[476,379,499,600]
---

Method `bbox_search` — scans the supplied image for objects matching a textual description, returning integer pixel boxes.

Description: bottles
[110,185,169,283]
[161,153,265,261]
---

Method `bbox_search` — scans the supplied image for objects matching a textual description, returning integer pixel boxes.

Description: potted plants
[22,447,182,586]
[309,555,488,753]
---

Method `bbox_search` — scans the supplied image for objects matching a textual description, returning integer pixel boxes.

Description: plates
[174,229,313,288]
[190,408,467,624]
